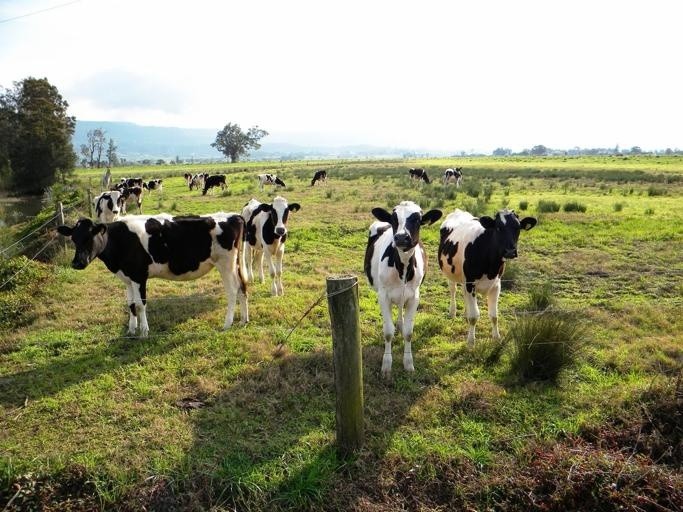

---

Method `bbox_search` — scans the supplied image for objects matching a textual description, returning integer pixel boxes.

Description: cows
[55,207,247,340]
[364,201,443,381]
[310,170,327,185]
[409,168,433,183]
[256,173,286,197]
[442,166,464,188]
[241,193,301,299]
[93,170,228,223]
[437,208,537,348]
